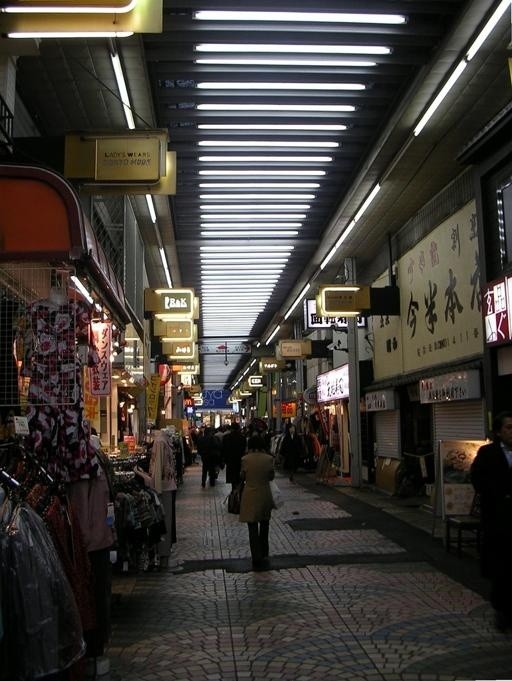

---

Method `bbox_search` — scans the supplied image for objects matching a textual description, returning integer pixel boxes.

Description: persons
[469,412,512,633]
[189,423,306,494]
[222,432,286,572]
[150,431,179,569]
[118,427,129,443]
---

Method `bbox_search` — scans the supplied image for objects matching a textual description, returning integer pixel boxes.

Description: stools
[446,516,482,556]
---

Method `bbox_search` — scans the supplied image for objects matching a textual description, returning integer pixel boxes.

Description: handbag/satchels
[228,494,241,514]
[269,479,283,510]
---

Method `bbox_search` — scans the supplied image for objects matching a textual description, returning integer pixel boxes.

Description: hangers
[0,267,149,517]
